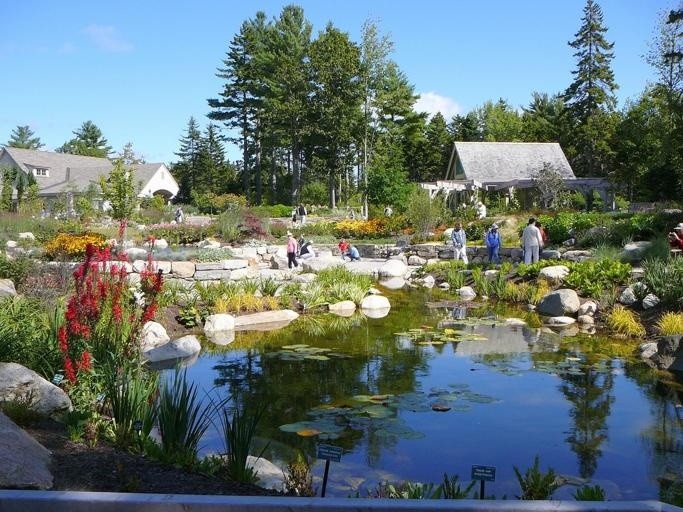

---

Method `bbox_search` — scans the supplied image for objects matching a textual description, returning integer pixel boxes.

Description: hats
[492,224,498,229]
[673,223,683,229]
[288,233,293,236]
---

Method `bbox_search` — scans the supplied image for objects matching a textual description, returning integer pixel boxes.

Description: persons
[668,222,683,251]
[298,235,315,261]
[451,223,468,265]
[522,218,547,266]
[175,207,184,223]
[288,202,307,224]
[287,232,300,270]
[486,223,502,265]
[384,206,393,218]
[338,238,360,262]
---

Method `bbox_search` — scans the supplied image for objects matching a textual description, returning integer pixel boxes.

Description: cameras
[669,233,674,237]
